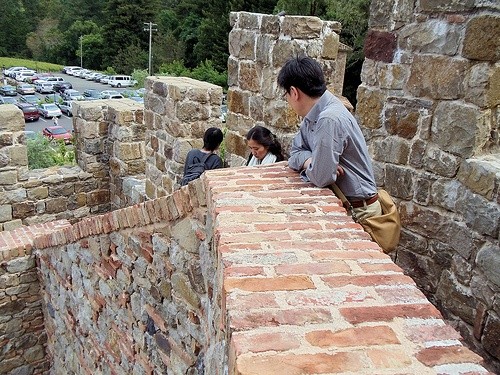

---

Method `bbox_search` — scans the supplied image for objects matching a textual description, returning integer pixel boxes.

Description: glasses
[280,89,291,102]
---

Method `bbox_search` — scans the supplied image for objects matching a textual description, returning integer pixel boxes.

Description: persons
[184,127,224,182]
[52,115,59,125]
[245,126,285,168]
[276,54,385,251]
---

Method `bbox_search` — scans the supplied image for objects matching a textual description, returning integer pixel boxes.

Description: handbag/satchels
[358,188,402,254]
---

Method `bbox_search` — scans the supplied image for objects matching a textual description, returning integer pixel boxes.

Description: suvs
[14,102,40,121]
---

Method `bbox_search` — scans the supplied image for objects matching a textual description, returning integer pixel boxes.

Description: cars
[37,104,62,119]
[42,125,73,145]
[219,94,228,123]
[0,66,147,104]
[58,100,76,117]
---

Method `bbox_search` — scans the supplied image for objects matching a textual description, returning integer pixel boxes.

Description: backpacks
[181,153,217,186]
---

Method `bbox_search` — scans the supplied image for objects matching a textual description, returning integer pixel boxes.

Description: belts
[350,192,378,208]
